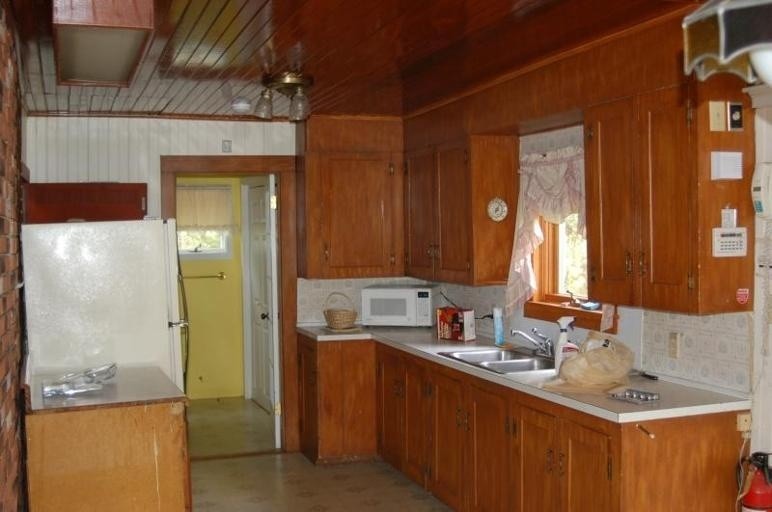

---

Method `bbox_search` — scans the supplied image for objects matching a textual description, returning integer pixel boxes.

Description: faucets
[510,328,553,355]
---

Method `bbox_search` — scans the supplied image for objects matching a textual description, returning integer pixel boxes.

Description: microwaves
[361,283,440,327]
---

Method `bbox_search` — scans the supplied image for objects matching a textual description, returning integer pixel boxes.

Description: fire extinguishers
[735,452,772,512]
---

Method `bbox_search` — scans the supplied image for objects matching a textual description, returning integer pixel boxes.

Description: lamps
[254,70,313,121]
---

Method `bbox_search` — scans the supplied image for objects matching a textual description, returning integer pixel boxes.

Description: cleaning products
[555,316,580,373]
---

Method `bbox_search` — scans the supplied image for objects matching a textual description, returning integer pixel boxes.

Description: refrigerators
[18,216,185,396]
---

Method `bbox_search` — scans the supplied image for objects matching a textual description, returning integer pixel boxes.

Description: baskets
[322,292,357,329]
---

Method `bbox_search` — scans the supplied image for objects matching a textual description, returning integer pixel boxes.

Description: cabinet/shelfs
[298,332,378,465]
[439,362,522,511]
[522,390,751,512]
[296,144,407,280]
[378,338,439,499]
[407,126,520,287]
[20,354,195,511]
[583,81,754,317]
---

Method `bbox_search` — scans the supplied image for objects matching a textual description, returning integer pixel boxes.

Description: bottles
[493,307,505,346]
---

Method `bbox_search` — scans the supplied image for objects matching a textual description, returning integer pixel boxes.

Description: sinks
[481,360,555,375]
[436,351,532,361]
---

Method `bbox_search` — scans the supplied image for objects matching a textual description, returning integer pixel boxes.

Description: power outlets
[491,303,498,318]
